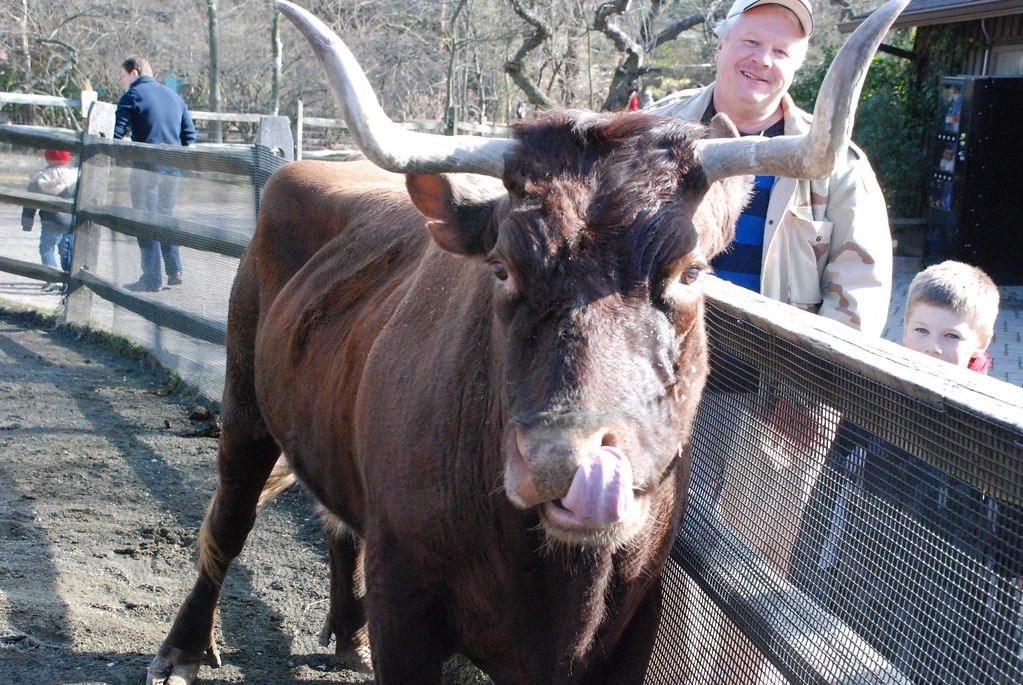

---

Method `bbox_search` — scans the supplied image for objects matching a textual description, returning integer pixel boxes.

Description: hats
[44,149,70,163]
[727,0,815,39]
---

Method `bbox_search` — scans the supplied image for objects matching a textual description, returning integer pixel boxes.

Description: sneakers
[40,281,64,293]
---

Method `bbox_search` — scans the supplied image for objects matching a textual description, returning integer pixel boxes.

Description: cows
[144,0,912,685]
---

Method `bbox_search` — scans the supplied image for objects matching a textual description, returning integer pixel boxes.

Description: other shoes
[167,272,182,285]
[122,281,163,293]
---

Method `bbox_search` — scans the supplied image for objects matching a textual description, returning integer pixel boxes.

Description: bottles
[926,173,962,211]
[938,87,961,132]
[936,133,968,175]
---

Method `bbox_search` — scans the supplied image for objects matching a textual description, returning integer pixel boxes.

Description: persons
[21,149,78,292]
[645,1,893,685]
[75,57,196,292]
[787,261,1000,685]
